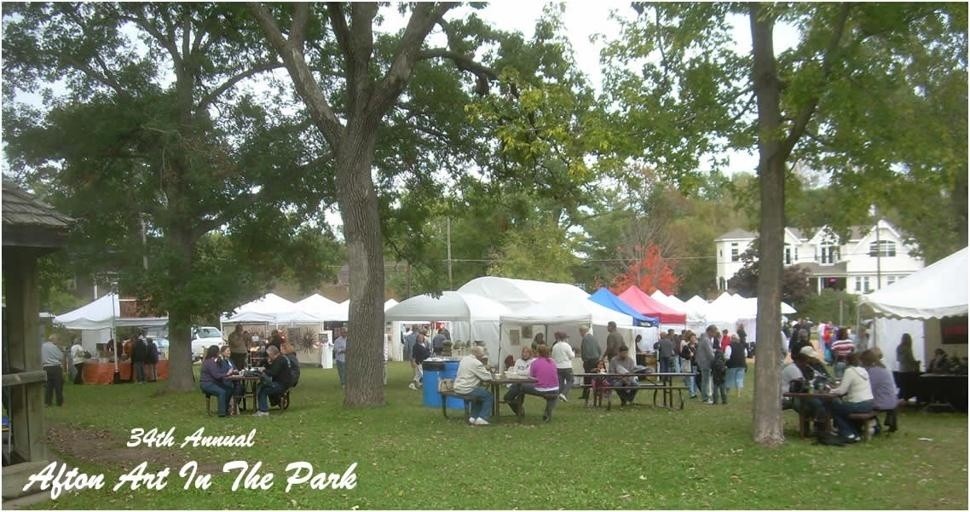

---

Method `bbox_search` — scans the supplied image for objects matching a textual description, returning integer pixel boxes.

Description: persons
[42,322,968,447]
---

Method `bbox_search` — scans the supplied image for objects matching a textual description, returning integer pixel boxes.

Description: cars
[140,337,169,361]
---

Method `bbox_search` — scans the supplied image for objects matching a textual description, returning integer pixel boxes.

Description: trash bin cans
[421,357,469,409]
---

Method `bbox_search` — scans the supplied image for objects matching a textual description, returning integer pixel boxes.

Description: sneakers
[559,393,568,402]
[689,394,728,406]
[408,380,422,389]
[251,410,268,416]
[469,416,491,424]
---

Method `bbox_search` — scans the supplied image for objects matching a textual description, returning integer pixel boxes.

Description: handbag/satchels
[679,357,692,373]
[790,377,808,391]
[439,379,453,392]
[77,349,92,358]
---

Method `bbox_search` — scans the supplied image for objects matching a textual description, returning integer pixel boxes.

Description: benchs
[202,388,218,416]
[598,387,691,410]
[579,383,666,408]
[523,390,558,424]
[850,408,894,444]
[439,389,479,425]
[267,389,293,413]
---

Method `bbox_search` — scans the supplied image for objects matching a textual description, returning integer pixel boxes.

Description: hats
[800,346,819,358]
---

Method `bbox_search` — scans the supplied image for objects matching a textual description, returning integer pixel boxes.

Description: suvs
[191,327,228,362]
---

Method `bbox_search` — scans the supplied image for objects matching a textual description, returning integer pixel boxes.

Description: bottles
[808,381,816,395]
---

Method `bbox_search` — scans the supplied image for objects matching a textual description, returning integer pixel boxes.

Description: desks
[783,392,839,439]
[920,372,968,413]
[224,372,262,415]
[574,373,701,408]
[490,375,539,418]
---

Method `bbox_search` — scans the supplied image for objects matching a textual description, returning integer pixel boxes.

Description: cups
[494,374,500,380]
